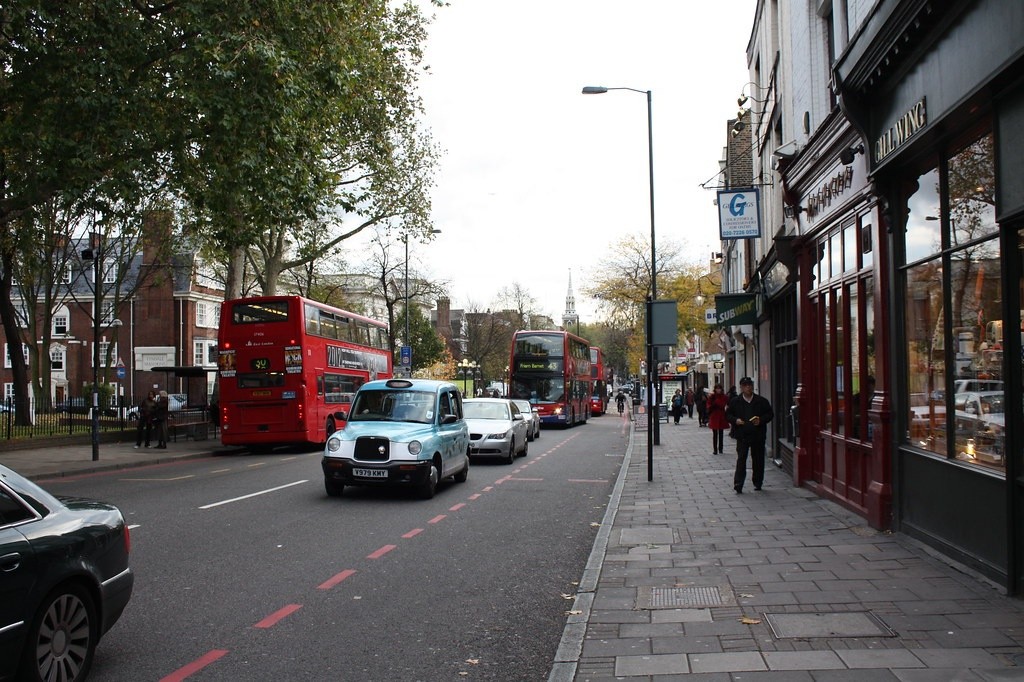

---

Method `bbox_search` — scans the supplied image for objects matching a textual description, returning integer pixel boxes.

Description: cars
[0,463,134,682]
[55,397,118,418]
[461,398,529,465]
[606,383,614,398]
[617,384,634,396]
[511,399,541,442]
[320,378,473,499]
[0,403,16,414]
[125,393,188,423]
[825,379,1005,468]
[486,387,500,398]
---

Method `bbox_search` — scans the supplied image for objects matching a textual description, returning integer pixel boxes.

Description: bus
[506,329,593,427]
[590,347,609,416]
[218,295,394,450]
[604,367,615,391]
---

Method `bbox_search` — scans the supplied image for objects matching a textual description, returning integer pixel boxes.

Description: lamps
[840,144,864,165]
[731,73,775,138]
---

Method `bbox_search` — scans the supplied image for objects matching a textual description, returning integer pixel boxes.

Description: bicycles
[615,399,625,417]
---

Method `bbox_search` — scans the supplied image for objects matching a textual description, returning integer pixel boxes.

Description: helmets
[159,390,168,398]
[618,391,623,396]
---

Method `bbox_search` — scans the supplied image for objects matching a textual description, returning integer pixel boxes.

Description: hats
[740,377,753,385]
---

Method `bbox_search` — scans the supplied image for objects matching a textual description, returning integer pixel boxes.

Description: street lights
[504,365,510,397]
[403,228,442,379]
[580,84,653,483]
[79,250,103,460]
[457,359,476,399]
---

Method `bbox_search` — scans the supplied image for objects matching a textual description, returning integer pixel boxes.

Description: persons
[726,384,737,438]
[685,385,708,427]
[476,386,499,398]
[362,393,384,414]
[672,389,684,424]
[516,382,530,398]
[615,392,626,412]
[706,384,728,455]
[961,366,998,391]
[726,378,775,493]
[134,391,168,449]
[211,401,220,427]
[853,376,874,442]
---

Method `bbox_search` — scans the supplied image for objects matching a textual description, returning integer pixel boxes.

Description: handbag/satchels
[702,392,707,401]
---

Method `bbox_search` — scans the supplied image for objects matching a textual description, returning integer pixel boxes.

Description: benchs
[168,420,218,443]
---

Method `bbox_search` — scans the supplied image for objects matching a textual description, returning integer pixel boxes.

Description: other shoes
[675,422,677,425]
[719,448,723,454]
[145,446,152,449]
[754,486,762,491]
[704,424,706,427]
[154,445,163,449]
[734,484,742,494]
[159,446,166,449]
[134,446,139,449]
[699,425,702,427]
[713,452,717,455]
[618,410,619,412]
[622,411,623,412]
